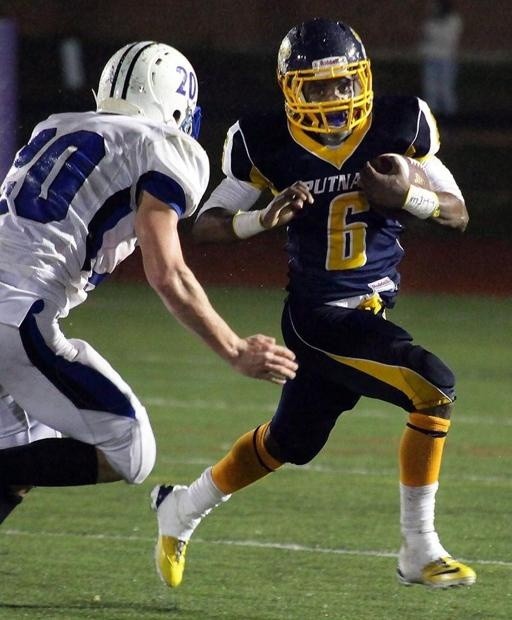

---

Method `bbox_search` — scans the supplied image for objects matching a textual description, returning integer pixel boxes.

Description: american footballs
[370,153,436,192]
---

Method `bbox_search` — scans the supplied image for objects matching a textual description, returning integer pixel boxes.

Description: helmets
[276,16,374,134]
[91,41,202,139]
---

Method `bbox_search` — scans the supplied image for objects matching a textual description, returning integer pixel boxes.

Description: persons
[0,40,303,523]
[146,25,477,594]
[418,0,464,116]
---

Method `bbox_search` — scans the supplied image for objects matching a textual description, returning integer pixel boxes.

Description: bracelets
[402,184,441,220]
[231,208,267,240]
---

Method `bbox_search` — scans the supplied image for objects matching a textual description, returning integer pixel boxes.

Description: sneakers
[150,482,194,587]
[396,544,476,589]
